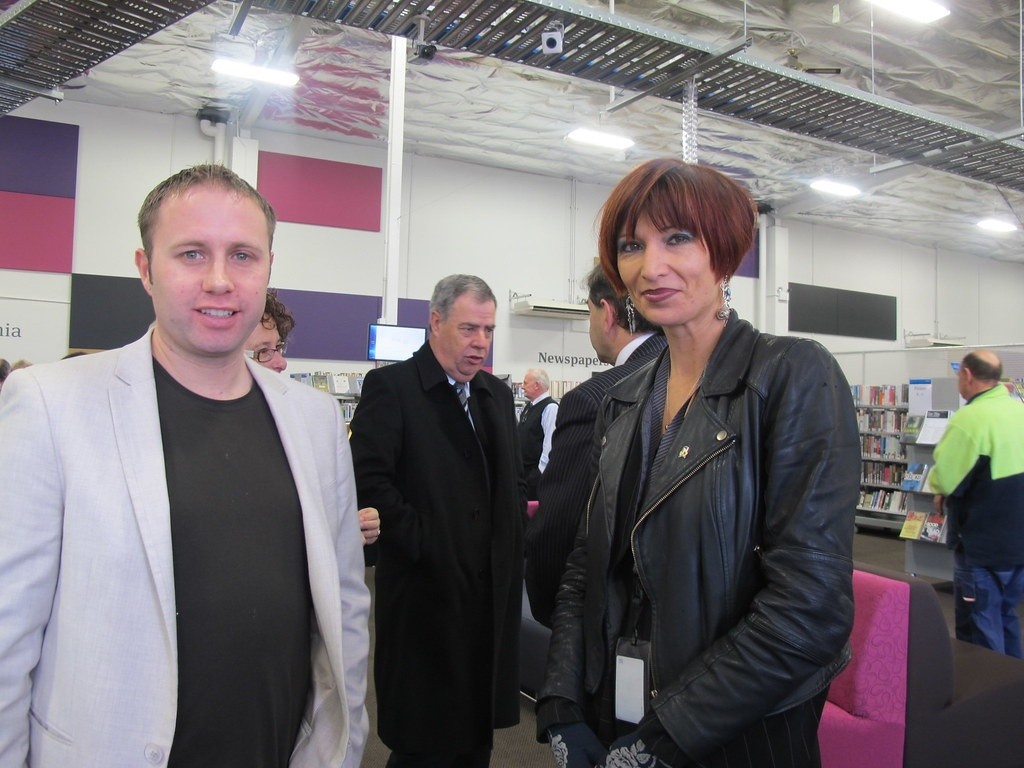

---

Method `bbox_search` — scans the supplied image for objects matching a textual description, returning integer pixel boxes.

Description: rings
[377,530,380,536]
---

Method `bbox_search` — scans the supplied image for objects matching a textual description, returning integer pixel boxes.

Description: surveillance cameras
[407,44,437,65]
[541,30,563,54]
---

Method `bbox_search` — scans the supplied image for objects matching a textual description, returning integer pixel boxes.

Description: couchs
[850,558,1024,767]
[507,497,907,768]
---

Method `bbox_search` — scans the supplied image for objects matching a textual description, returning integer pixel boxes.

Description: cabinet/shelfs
[291,368,369,441]
[496,376,581,422]
[847,385,908,537]
[901,381,967,586]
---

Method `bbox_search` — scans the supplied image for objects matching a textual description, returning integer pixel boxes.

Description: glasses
[243,341,287,363]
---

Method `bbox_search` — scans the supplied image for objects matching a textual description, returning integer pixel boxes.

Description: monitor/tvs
[367,324,427,361]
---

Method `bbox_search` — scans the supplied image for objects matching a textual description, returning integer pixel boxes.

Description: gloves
[545,723,604,768]
[602,732,669,768]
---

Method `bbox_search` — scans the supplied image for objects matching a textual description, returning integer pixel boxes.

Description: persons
[353,274,523,765]
[239,292,380,545]
[0,165,374,768]
[535,155,863,764]
[515,369,559,541]
[524,258,667,630]
[927,352,1024,663]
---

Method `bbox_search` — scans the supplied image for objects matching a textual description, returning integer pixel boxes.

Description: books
[845,379,949,545]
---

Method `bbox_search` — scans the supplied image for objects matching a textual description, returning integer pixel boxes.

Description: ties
[451,381,476,432]
